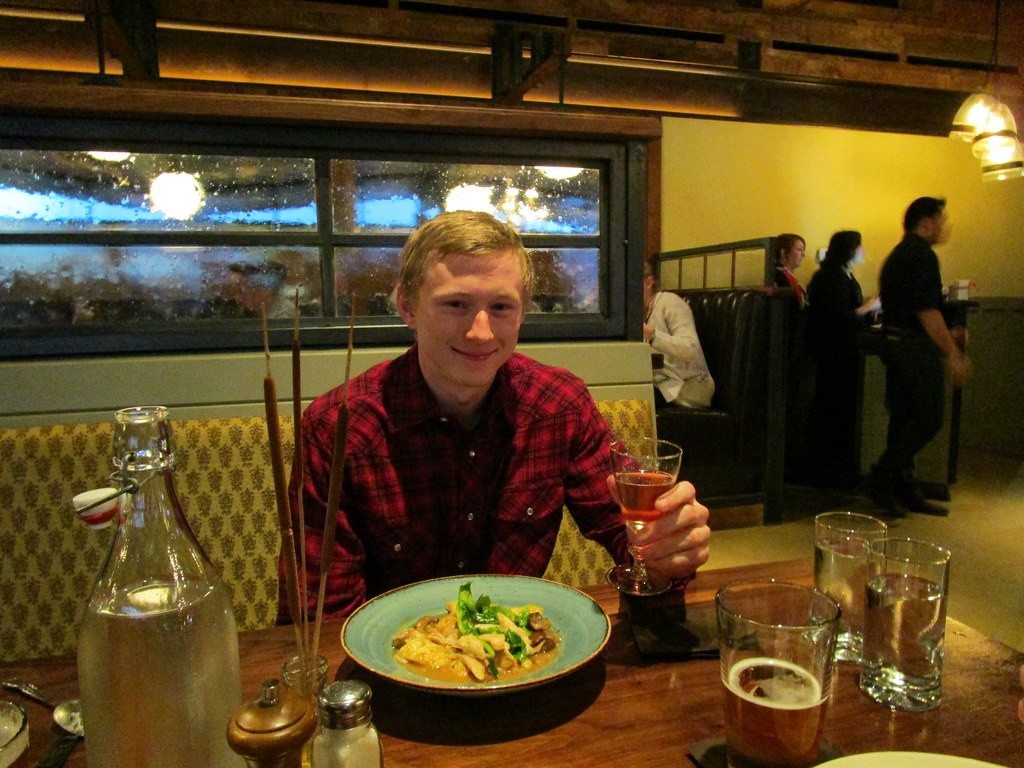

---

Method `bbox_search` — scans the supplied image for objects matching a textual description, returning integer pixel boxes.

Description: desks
[0,558,1024,768]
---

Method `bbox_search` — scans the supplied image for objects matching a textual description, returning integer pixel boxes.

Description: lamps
[949,0,1024,183]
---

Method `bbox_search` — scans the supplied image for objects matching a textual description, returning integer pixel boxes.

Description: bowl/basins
[341,574,611,697]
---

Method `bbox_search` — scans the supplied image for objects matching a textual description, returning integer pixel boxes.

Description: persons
[644,251,714,410]
[274,210,712,626]
[774,198,973,517]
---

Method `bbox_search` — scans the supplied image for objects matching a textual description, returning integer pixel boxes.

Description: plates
[812,751,1011,768]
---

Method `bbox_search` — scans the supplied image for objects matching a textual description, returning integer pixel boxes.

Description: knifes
[34,731,78,768]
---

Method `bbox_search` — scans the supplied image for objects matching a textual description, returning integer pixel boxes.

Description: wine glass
[606,439,683,596]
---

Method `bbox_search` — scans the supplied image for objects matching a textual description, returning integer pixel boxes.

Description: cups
[815,512,888,665]
[0,700,29,768]
[859,539,953,712]
[716,579,843,768]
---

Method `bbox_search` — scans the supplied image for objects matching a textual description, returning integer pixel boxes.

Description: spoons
[2,676,86,736]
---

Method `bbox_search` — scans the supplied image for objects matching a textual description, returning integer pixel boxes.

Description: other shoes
[864,472,949,518]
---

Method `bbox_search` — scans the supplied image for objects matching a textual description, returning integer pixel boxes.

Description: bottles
[282,654,328,768]
[312,681,385,768]
[78,407,245,768]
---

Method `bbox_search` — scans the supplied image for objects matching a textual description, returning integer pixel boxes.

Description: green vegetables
[456,581,535,679]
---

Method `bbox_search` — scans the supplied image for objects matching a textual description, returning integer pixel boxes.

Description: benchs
[0,397,658,664]
[655,290,770,531]
